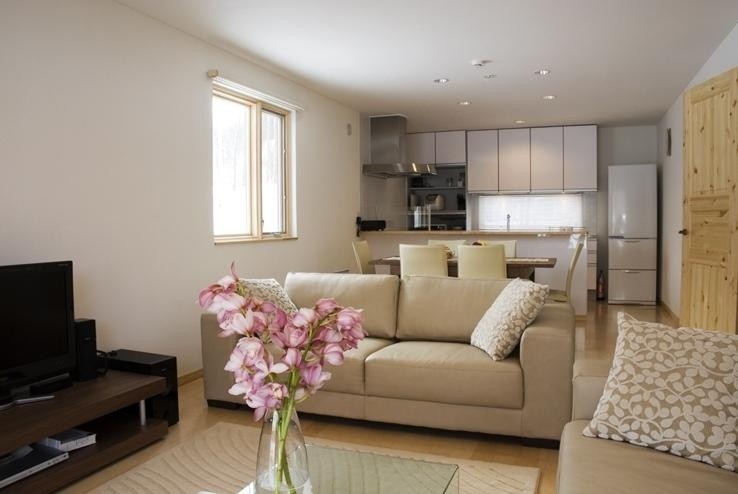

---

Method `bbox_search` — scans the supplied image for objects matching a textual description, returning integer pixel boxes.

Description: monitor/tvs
[0,260,78,411]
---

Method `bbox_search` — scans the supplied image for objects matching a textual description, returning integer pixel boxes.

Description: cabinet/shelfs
[1,362,172,493]
[404,161,479,232]
[404,128,467,168]
[529,122,599,195]
[587,235,598,304]
[465,125,531,197]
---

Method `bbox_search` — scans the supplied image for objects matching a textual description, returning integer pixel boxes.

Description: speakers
[73,318,98,370]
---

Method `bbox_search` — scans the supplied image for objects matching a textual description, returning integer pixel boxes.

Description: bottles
[447,177,452,187]
[457,175,463,187]
[409,190,418,212]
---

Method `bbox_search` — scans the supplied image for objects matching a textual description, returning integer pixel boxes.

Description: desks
[369,253,556,282]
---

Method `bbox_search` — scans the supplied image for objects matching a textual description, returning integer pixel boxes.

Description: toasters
[424,194,445,211]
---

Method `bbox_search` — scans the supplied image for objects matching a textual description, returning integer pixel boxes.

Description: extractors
[361,111,438,179]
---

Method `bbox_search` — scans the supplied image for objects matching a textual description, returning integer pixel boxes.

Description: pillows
[238,277,299,343]
[468,274,550,362]
[580,308,736,474]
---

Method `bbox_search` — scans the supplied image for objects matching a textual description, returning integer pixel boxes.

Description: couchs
[555,351,736,493]
[199,268,576,449]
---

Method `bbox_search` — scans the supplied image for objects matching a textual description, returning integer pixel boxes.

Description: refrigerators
[608,164,657,306]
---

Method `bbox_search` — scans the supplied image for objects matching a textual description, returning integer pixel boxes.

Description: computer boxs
[98,348,180,427]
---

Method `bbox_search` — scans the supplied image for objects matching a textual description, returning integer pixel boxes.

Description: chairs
[427,238,466,258]
[540,238,583,306]
[351,239,378,274]
[477,240,518,259]
[398,243,450,278]
[456,244,508,280]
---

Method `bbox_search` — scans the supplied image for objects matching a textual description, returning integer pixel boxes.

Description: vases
[253,389,311,494]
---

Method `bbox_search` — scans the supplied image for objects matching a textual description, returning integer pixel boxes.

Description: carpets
[84,415,542,493]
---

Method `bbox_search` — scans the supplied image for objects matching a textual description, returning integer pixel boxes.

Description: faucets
[506,213,511,230]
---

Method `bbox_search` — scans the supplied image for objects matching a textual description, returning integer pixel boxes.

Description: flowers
[193,259,368,494]
